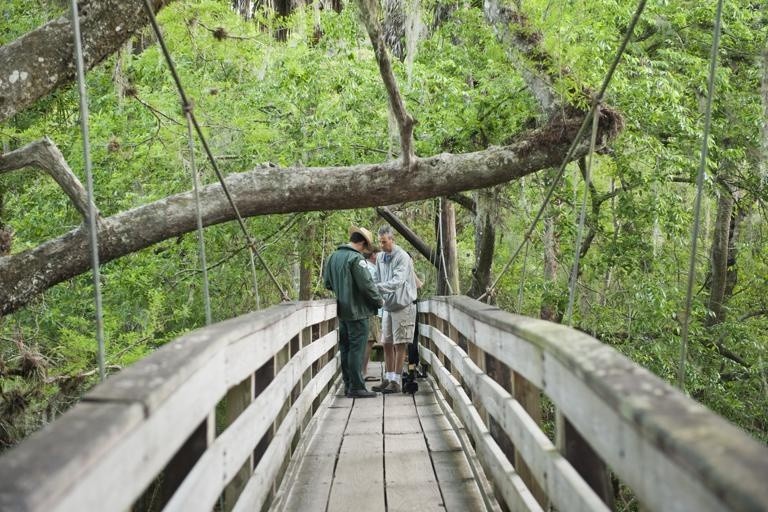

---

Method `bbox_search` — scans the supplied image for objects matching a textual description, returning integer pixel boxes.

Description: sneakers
[344,376,400,398]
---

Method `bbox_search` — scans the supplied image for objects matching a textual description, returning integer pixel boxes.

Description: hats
[348,226,374,254]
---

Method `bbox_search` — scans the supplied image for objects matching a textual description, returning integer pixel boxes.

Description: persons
[362,224,423,395]
[323,225,383,399]
